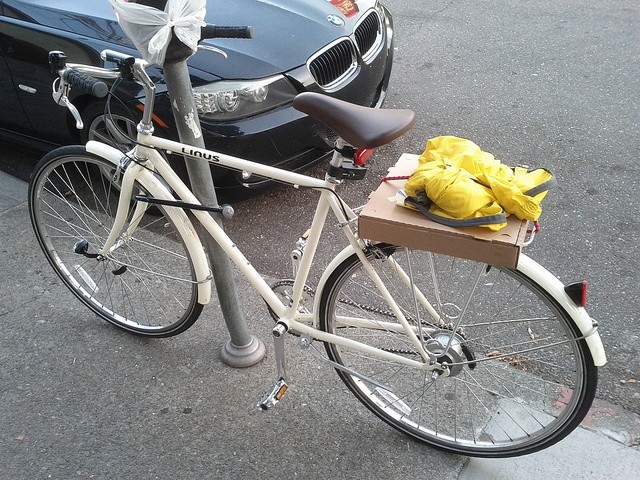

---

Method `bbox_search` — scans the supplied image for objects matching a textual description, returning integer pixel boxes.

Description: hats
[0,0,398,218]
